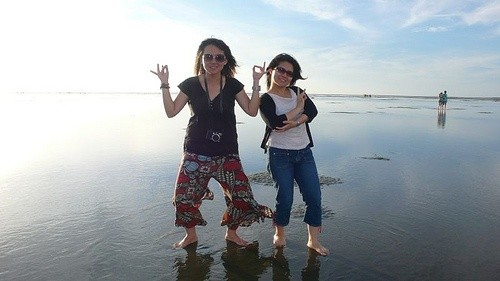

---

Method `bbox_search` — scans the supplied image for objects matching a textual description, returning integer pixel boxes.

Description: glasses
[201,54,226,62]
[272,67,294,77]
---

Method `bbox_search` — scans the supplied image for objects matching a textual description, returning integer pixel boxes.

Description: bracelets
[252,86,261,92]
[161,82,171,89]
[296,119,300,126]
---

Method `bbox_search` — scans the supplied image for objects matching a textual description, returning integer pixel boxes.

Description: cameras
[206,129,224,143]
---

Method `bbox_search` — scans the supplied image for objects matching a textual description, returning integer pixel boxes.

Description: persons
[259,54,329,256]
[439,91,448,108]
[150,38,268,250]
